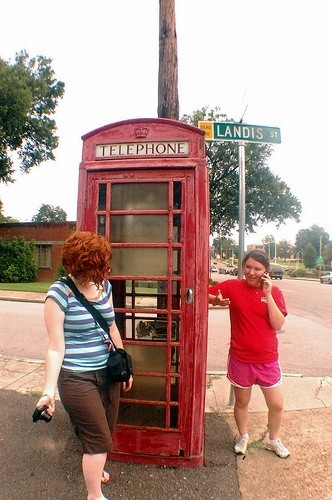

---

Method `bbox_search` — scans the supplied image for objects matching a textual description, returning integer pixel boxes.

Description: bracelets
[40,394,52,397]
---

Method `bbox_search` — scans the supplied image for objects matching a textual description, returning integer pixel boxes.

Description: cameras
[33,405,52,423]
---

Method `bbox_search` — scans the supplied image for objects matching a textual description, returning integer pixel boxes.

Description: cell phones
[259,272,267,283]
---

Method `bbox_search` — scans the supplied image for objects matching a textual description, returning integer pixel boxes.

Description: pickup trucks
[269,268,283,280]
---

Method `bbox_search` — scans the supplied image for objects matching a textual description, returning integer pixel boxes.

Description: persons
[206,250,291,459]
[36,231,134,500]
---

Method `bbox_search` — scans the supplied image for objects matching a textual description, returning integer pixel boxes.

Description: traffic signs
[198,121,281,144]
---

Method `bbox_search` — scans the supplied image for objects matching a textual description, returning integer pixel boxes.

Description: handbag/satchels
[106,348,133,383]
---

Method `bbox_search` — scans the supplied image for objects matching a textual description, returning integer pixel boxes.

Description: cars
[212,266,238,276]
[320,273,332,283]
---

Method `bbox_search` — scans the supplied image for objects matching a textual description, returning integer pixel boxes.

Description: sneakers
[264,432,290,459]
[234,433,249,454]
